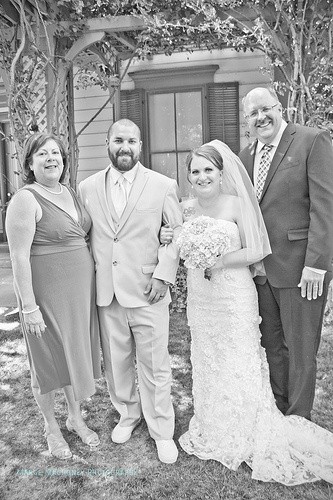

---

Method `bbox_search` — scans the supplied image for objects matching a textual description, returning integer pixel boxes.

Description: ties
[255,145,273,203]
[114,176,127,217]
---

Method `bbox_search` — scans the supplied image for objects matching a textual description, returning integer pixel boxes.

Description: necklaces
[34,181,63,195]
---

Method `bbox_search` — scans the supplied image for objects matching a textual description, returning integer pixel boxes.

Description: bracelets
[22,306,39,315]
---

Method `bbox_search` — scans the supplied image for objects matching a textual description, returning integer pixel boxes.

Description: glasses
[246,101,279,120]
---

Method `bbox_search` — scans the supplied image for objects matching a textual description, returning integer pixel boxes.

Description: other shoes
[111,417,141,443]
[155,438,179,464]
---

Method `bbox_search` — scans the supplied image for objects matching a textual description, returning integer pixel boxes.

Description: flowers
[177,215,231,281]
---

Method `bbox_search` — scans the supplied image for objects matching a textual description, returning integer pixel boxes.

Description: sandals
[66,418,100,447]
[43,427,72,460]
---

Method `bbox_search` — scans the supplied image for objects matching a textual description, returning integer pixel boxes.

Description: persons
[159,141,276,472]
[6,133,102,460]
[238,87,333,421]
[77,120,183,465]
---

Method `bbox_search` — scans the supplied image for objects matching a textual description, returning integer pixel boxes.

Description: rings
[160,296,163,297]
